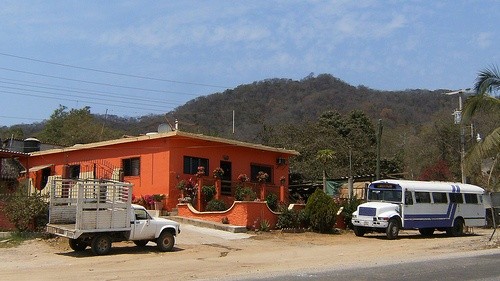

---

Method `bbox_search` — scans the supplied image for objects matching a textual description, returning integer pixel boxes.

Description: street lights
[444,89,467,184]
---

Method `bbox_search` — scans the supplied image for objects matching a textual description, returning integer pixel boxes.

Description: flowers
[184,177,196,191]
[279,176,287,183]
[237,173,250,183]
[213,166,224,179]
[122,191,165,206]
[194,166,205,178]
[256,171,269,182]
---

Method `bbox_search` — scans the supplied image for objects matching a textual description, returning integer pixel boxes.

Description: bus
[350,179,488,241]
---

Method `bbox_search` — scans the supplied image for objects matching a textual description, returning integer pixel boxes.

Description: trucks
[44,175,182,256]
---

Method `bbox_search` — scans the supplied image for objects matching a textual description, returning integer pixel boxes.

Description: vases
[145,204,150,210]
[154,202,163,210]
[150,204,155,210]
